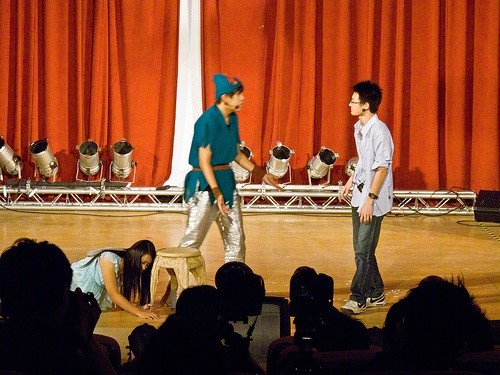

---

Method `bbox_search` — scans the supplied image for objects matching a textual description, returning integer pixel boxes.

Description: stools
[151,248,208,311]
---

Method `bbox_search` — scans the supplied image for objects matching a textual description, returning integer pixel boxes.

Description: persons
[145,74,282,264]
[0,238,500,375]
[70,240,160,320]
[340,80,395,314]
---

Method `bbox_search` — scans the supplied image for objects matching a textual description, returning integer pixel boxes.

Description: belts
[193,163,231,172]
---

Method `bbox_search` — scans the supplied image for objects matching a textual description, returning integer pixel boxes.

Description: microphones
[362,109,370,112]
[224,102,238,109]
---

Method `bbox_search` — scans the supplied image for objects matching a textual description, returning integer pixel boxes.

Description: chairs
[267,321,499,374]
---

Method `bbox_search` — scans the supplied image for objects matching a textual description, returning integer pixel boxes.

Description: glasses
[350,100,362,105]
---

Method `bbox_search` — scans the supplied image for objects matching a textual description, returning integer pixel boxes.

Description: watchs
[368,192,378,200]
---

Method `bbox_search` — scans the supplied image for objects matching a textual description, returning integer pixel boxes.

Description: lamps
[230,140,252,185]
[109,138,138,183]
[306,144,340,185]
[265,140,295,185]
[0,136,26,180]
[76,138,104,182]
[30,137,58,184]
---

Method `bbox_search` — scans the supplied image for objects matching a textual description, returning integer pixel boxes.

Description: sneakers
[365,293,386,308]
[340,299,367,315]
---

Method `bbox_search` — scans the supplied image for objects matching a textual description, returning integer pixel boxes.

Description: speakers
[474,190,500,223]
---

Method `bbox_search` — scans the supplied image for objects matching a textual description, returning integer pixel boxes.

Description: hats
[213,73,242,99]
